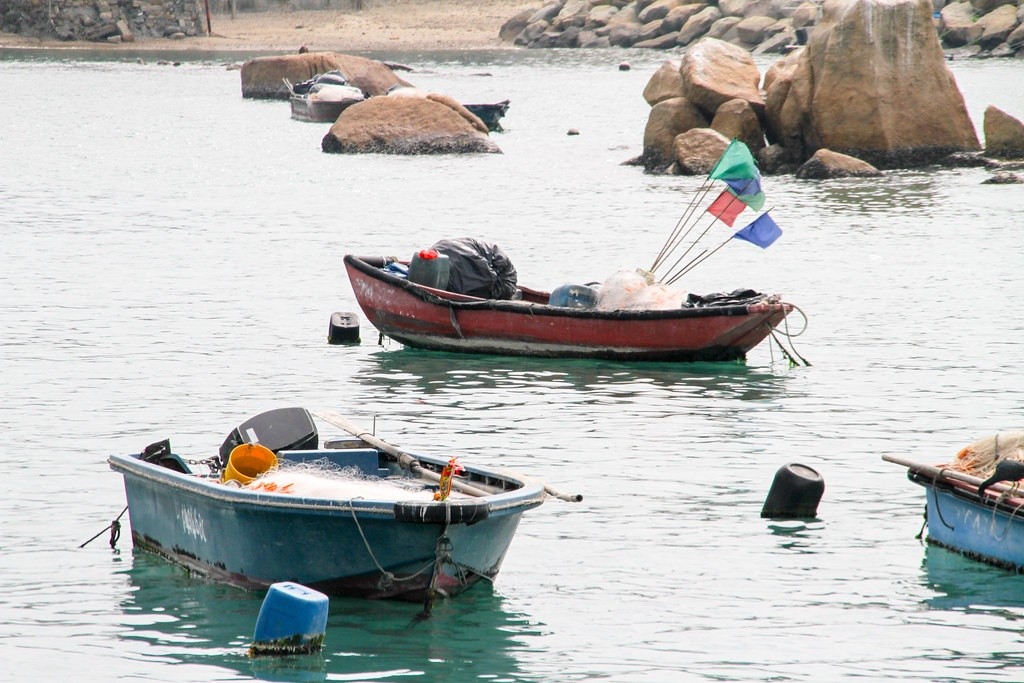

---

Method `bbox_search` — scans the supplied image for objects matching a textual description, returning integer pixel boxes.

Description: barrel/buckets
[549,284,599,310]
[326,311,362,346]
[250,581,328,658]
[224,442,278,487]
[760,460,825,521]
[406,251,451,293]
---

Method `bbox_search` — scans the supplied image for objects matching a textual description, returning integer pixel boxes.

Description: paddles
[311,408,492,501]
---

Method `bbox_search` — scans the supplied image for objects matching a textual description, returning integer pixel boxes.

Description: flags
[707,191,746,227]
[709,140,765,211]
[735,214,782,249]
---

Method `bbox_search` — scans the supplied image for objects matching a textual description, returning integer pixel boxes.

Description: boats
[290,72,368,122]
[907,431,1024,570]
[344,255,793,359]
[107,410,547,601]
[463,100,511,132]
